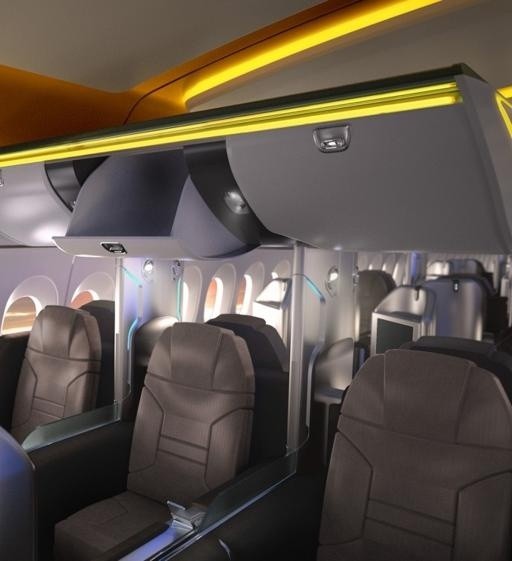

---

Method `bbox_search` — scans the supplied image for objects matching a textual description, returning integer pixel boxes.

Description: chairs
[317,349,512,561]
[52,322,257,561]
[11,304,102,445]
